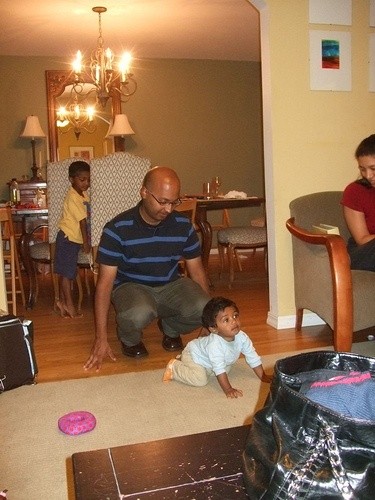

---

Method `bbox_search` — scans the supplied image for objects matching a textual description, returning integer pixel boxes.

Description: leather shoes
[120,340,149,358]
[162,333,183,352]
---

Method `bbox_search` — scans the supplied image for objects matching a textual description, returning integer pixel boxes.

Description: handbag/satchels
[0,313,39,394]
[241,350,375,500]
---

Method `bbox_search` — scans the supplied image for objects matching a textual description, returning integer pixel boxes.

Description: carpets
[0,339,375,500]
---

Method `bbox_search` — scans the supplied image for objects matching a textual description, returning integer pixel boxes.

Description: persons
[82,166,212,371]
[338,134,375,273]
[162,297,272,399]
[53,161,90,318]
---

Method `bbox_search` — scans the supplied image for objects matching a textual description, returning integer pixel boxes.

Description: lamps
[109,114,136,152]
[72,7,138,111]
[19,115,46,180]
[57,93,97,142]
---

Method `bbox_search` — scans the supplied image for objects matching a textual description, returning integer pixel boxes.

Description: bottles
[9,178,20,205]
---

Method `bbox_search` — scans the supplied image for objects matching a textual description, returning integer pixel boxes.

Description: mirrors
[45,68,126,163]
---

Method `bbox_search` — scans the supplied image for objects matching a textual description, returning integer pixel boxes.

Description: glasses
[145,186,182,207]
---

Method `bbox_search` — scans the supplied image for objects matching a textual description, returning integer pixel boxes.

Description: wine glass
[211,176,222,199]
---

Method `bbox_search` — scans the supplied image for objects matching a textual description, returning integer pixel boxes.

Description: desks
[183,195,266,289]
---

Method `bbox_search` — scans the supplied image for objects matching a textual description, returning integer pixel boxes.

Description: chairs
[285,190,375,353]
[0,155,272,316]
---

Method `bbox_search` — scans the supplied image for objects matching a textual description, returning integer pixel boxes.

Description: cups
[203,182,213,200]
